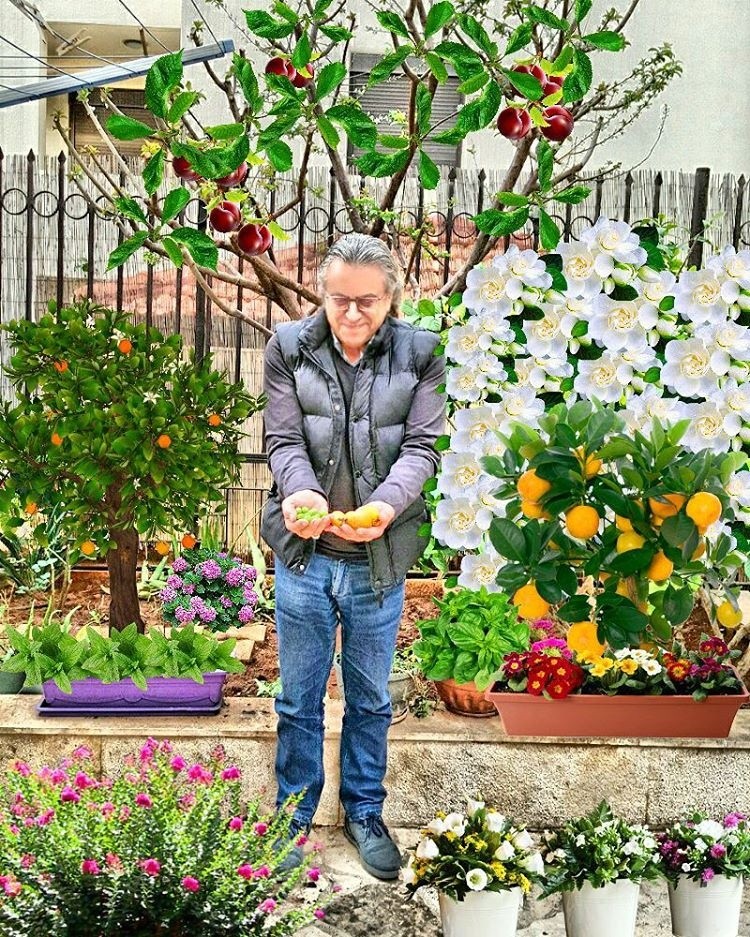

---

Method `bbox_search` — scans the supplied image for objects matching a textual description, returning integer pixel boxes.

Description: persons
[261,233,446,883]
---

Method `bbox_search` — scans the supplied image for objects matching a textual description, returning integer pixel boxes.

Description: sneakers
[343,812,403,878]
[268,822,309,875]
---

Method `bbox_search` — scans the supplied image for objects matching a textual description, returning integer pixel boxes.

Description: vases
[560,870,641,937]
[667,870,743,937]
[486,681,749,737]
[156,626,268,664]
[436,889,522,937]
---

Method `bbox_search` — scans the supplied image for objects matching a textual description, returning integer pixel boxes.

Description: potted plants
[2,624,242,715]
[414,587,528,719]
[338,653,427,724]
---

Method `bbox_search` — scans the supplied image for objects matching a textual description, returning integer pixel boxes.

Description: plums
[266,56,314,88]
[172,151,272,256]
[499,60,575,141]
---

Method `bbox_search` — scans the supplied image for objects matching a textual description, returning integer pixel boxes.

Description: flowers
[503,613,742,704]
[659,812,750,888]
[404,796,543,902]
[165,546,259,628]
[544,801,662,888]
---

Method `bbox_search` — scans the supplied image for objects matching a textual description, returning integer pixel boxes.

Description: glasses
[324,287,388,312]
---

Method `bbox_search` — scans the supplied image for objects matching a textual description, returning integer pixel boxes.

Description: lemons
[515,447,744,658]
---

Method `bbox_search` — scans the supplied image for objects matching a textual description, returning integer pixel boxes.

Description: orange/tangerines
[22,340,222,556]
